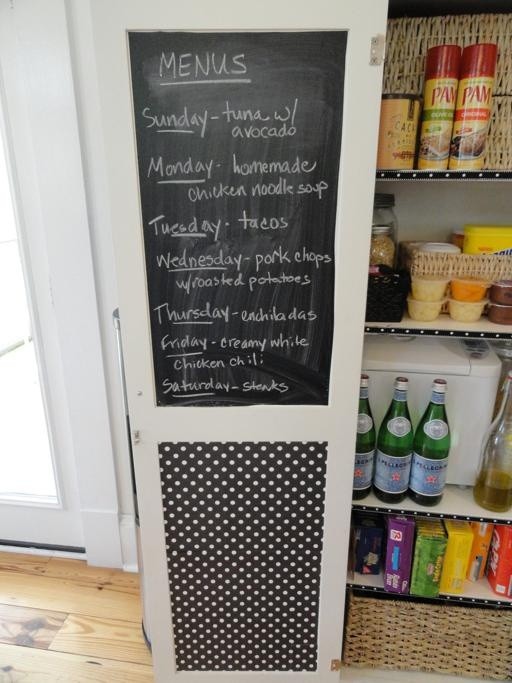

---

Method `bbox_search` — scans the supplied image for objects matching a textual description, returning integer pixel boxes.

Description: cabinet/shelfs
[341,0,512,607]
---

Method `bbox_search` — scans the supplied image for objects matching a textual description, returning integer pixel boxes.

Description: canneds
[370,226,398,277]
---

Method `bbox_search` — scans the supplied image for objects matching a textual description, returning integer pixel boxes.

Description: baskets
[343,590,512,682]
[383,14,512,170]
[397,241,512,281]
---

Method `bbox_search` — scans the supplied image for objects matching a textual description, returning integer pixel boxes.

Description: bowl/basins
[406,273,511,326]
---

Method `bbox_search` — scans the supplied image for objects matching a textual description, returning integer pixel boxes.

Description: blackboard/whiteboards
[126,28,348,405]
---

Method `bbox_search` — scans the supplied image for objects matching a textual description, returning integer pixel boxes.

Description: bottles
[449,41,498,170]
[370,222,397,269]
[407,377,450,507]
[351,373,377,501]
[471,368,512,513]
[418,44,462,170]
[373,376,415,505]
[370,191,400,269]
